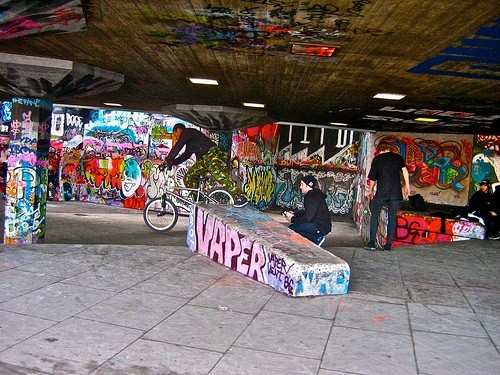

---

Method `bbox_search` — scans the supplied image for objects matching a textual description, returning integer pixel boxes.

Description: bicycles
[142,162,237,232]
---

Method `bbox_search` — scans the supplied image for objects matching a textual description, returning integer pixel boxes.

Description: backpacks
[409,194,427,212]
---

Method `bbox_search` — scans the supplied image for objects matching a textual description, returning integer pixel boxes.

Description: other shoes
[364,244,376,250]
[384,247,390,252]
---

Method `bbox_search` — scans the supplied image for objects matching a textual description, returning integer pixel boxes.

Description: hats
[481,179,492,189]
[301,176,317,188]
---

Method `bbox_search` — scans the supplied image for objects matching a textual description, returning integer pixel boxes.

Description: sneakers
[488,234,500,241]
[234,197,248,208]
[314,236,326,247]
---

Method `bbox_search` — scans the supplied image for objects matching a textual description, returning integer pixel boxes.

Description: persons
[282,175,332,246]
[363,143,410,252]
[469,179,500,240]
[157,123,248,208]
